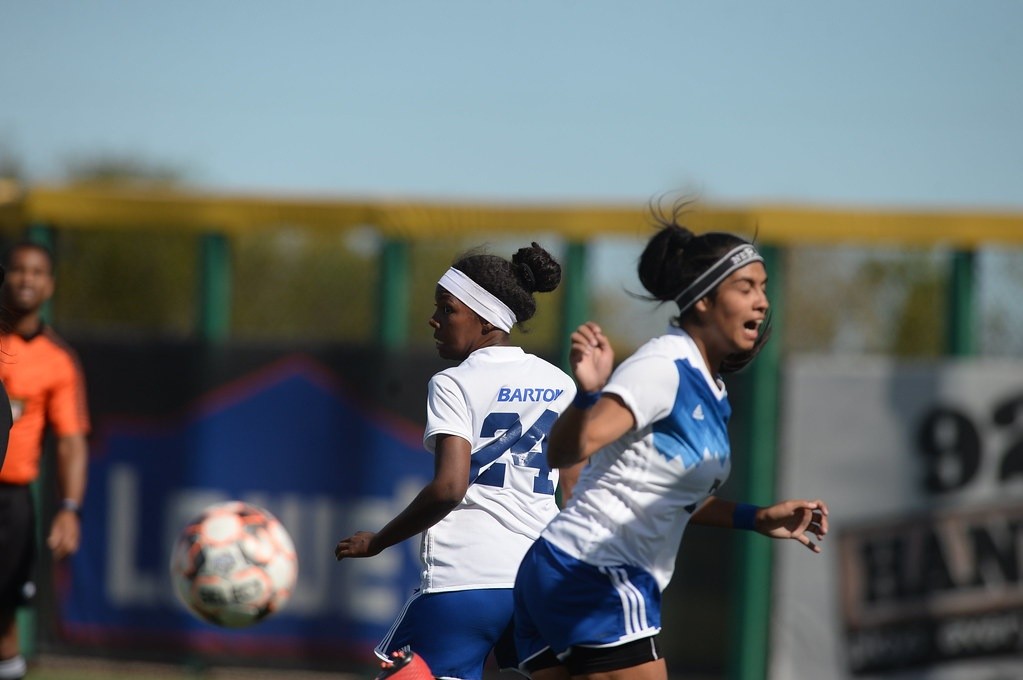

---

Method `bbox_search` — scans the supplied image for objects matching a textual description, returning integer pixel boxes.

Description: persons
[0,238,88,680]
[334,223,829,680]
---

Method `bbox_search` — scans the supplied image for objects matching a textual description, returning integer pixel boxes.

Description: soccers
[173,501,301,629]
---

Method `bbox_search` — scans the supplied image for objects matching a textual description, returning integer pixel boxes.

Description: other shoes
[0,655,26,679]
[372,645,435,680]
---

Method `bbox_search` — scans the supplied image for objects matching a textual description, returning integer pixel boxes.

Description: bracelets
[59,500,80,517]
